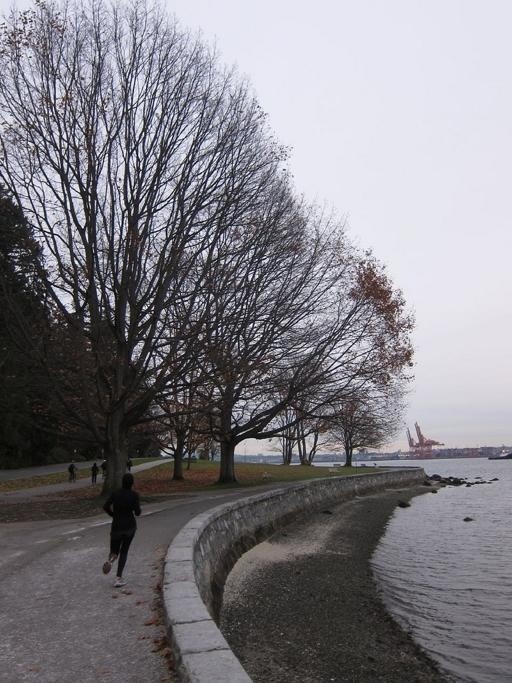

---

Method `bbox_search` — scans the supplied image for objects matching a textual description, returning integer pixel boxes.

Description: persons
[101,462,106,472]
[91,463,99,488]
[103,473,141,586]
[127,457,134,471]
[68,464,77,480]
